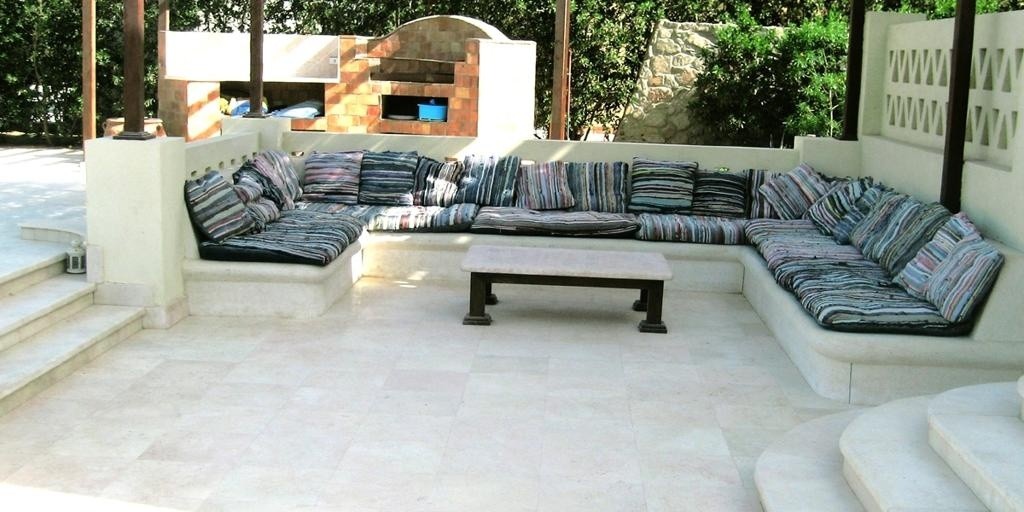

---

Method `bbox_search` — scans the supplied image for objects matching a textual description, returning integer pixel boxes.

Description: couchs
[181,155,1024,407]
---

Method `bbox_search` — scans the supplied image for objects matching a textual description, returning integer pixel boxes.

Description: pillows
[807,175,873,236]
[411,156,465,208]
[358,150,419,206]
[626,156,699,216]
[454,154,521,207]
[564,161,629,213]
[758,161,833,221]
[922,232,1004,324]
[232,150,305,224]
[184,170,256,245]
[830,183,895,245]
[750,161,783,219]
[892,211,981,301]
[848,191,927,262]
[691,168,754,221]
[515,160,576,210]
[878,201,954,277]
[300,151,363,205]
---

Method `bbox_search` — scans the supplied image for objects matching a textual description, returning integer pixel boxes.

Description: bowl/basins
[418,103,449,122]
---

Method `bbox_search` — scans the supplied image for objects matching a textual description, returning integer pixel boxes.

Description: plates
[387,114,414,122]
